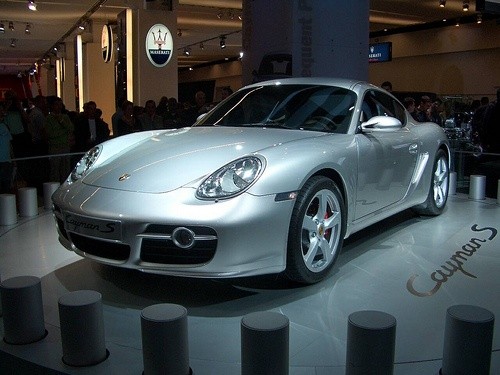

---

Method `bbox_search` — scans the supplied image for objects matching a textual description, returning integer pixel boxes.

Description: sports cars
[50,75,454,285]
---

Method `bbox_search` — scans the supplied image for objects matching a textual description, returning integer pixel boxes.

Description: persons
[381,81,500,131]
[0,86,237,194]
